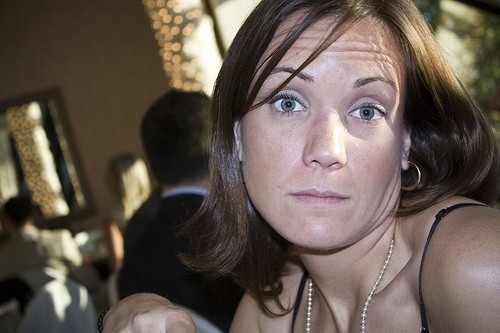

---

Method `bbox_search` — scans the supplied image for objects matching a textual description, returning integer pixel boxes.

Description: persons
[101,0,500,333]
[0,195,98,333]
[115,90,238,333]
[103,151,151,269]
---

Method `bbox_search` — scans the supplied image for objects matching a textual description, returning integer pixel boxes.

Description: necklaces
[305,239,394,333]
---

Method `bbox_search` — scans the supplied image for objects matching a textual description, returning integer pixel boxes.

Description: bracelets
[97,307,110,333]
